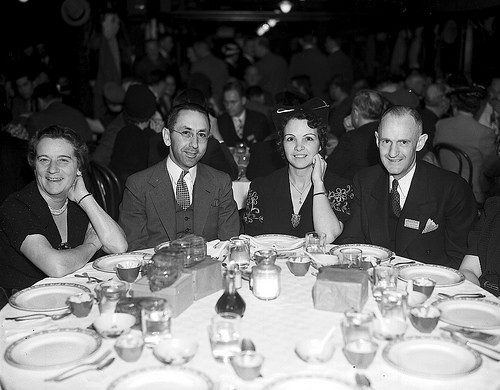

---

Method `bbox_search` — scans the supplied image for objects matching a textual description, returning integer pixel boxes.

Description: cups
[94,280,173,349]
[305,231,397,302]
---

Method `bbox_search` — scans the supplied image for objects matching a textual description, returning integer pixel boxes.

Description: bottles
[151,234,280,316]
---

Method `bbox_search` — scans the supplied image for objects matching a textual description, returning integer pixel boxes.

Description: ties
[175,170,190,210]
[390,179,401,218]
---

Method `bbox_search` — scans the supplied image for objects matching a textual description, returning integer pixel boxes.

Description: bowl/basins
[289,258,311,276]
[115,259,142,282]
[342,277,440,369]
[93,313,264,380]
[68,292,93,318]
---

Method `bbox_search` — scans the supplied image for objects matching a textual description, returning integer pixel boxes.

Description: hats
[378,88,419,108]
[125,84,155,119]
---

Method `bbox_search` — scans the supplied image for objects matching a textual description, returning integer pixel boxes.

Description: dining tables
[0,236,499,390]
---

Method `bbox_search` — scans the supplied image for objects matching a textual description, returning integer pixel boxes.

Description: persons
[0,35,500,206]
[459,196,500,300]
[0,124,128,310]
[339,107,477,270]
[117,102,240,253]
[243,107,355,243]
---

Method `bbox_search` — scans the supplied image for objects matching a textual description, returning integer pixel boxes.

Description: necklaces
[48,199,67,215]
[290,180,310,205]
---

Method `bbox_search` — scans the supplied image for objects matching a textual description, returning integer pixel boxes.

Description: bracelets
[312,192,327,197]
[77,193,92,205]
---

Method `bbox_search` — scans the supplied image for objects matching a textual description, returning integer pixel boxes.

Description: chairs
[89,161,122,222]
[433,141,474,191]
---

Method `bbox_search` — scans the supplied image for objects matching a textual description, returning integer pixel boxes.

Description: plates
[431,298,500,332]
[154,241,173,252]
[330,244,392,262]
[93,252,153,272]
[397,264,465,287]
[383,335,482,377]
[105,363,214,390]
[250,234,305,253]
[262,374,358,390]
[4,283,102,369]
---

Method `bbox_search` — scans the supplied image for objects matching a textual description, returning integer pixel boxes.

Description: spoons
[83,272,95,283]
[242,338,263,378]
[56,358,115,382]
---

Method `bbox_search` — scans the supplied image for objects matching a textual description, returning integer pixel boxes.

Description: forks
[44,347,111,381]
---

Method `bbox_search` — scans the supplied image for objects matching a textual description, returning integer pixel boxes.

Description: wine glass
[342,309,380,389]
[230,144,249,181]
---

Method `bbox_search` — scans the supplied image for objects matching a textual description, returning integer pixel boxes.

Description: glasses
[171,127,208,142]
